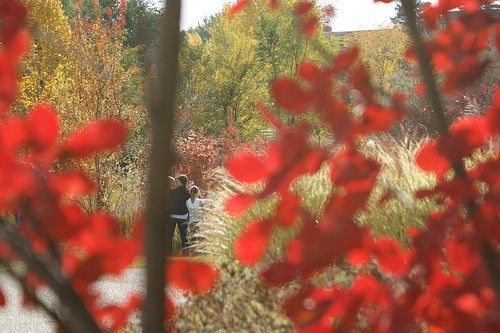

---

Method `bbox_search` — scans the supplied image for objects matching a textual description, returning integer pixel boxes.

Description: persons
[186,185,204,241]
[168,175,191,255]
[168,175,176,192]
